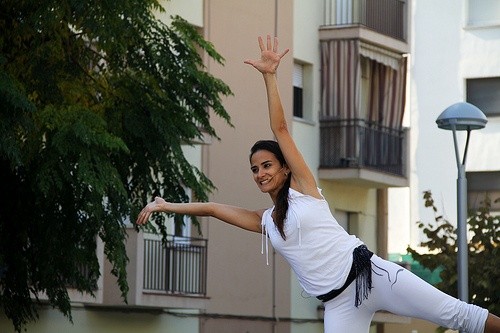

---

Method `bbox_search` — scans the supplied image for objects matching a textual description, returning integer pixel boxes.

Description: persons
[135,33,500,333]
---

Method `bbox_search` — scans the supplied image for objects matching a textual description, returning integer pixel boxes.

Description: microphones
[268,168,284,180]
